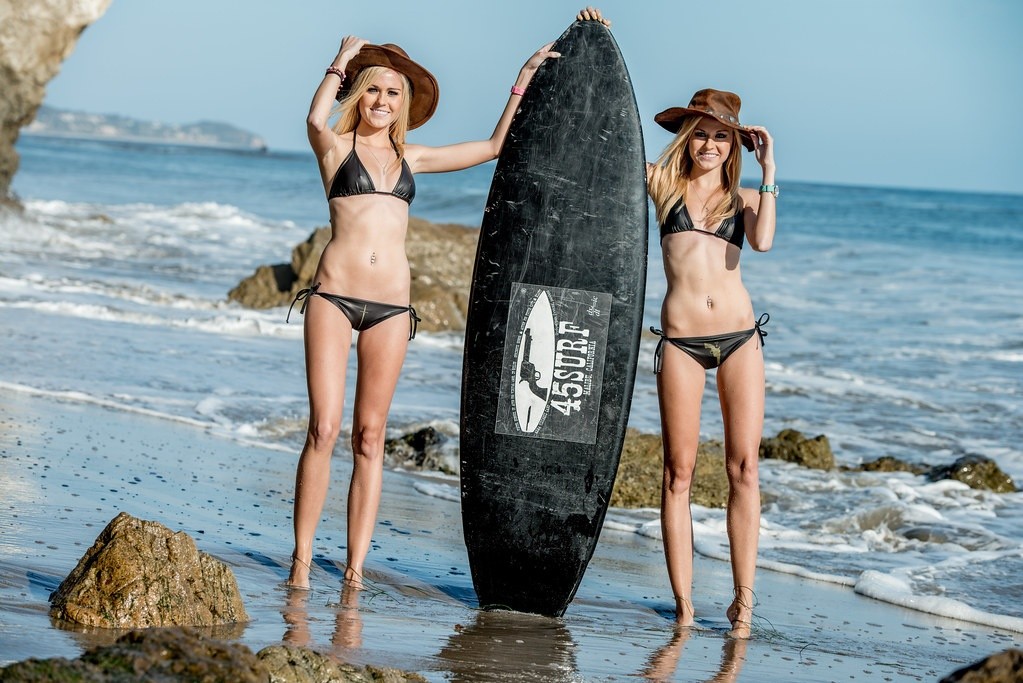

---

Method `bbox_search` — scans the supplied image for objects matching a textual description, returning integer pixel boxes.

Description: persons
[576,5,778,641]
[284,35,560,590]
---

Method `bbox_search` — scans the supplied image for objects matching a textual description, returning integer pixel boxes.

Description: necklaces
[686,173,725,213]
[356,129,392,175]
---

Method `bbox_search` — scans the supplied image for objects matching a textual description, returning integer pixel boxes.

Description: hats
[655,88,760,152]
[335,44,439,131]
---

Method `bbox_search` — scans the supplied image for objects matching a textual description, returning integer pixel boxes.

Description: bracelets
[510,85,526,96]
[325,66,347,81]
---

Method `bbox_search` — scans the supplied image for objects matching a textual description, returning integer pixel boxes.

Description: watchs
[758,185,780,198]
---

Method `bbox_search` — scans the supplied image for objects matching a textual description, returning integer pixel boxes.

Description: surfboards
[458,12,649,621]
